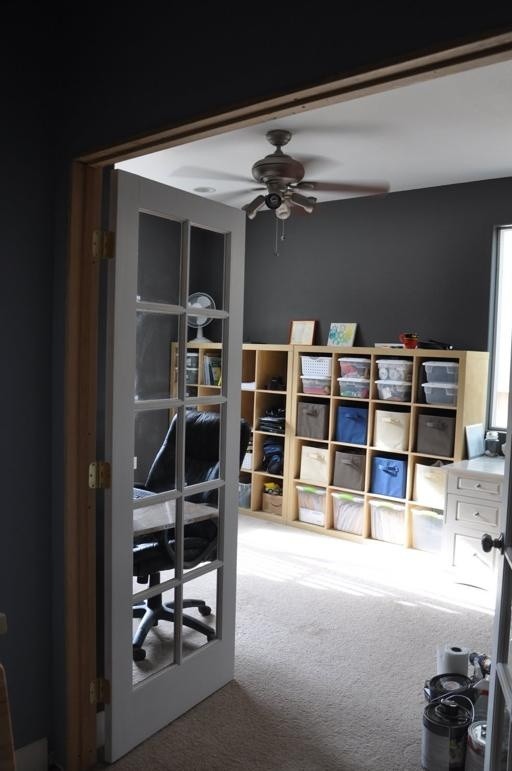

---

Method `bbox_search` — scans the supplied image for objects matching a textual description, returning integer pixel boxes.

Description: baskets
[300,356,333,378]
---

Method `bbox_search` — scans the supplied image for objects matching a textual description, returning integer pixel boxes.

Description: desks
[132,487,219,537]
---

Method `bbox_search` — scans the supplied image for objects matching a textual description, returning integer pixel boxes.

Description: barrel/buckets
[423,672,476,711]
[420,694,475,771]
[465,720,487,771]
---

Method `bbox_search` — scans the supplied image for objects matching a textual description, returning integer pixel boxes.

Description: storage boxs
[297,354,459,552]
[237,477,251,510]
[261,491,283,516]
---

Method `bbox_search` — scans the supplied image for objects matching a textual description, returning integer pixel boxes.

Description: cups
[485,440,500,457]
[403,333,417,350]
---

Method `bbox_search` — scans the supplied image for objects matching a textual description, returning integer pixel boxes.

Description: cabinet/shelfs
[289,343,490,556]
[440,454,506,592]
[167,339,292,526]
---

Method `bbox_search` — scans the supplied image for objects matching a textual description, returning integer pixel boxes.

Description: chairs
[133,407,252,662]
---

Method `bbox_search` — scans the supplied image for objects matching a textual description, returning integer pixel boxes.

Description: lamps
[242,177,317,219]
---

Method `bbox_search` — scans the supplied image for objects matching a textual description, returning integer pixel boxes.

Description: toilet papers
[442,643,470,677]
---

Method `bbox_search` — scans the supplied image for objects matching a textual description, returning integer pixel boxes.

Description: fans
[187,291,218,343]
[173,131,389,219]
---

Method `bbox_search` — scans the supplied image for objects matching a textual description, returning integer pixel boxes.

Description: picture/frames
[287,320,316,346]
